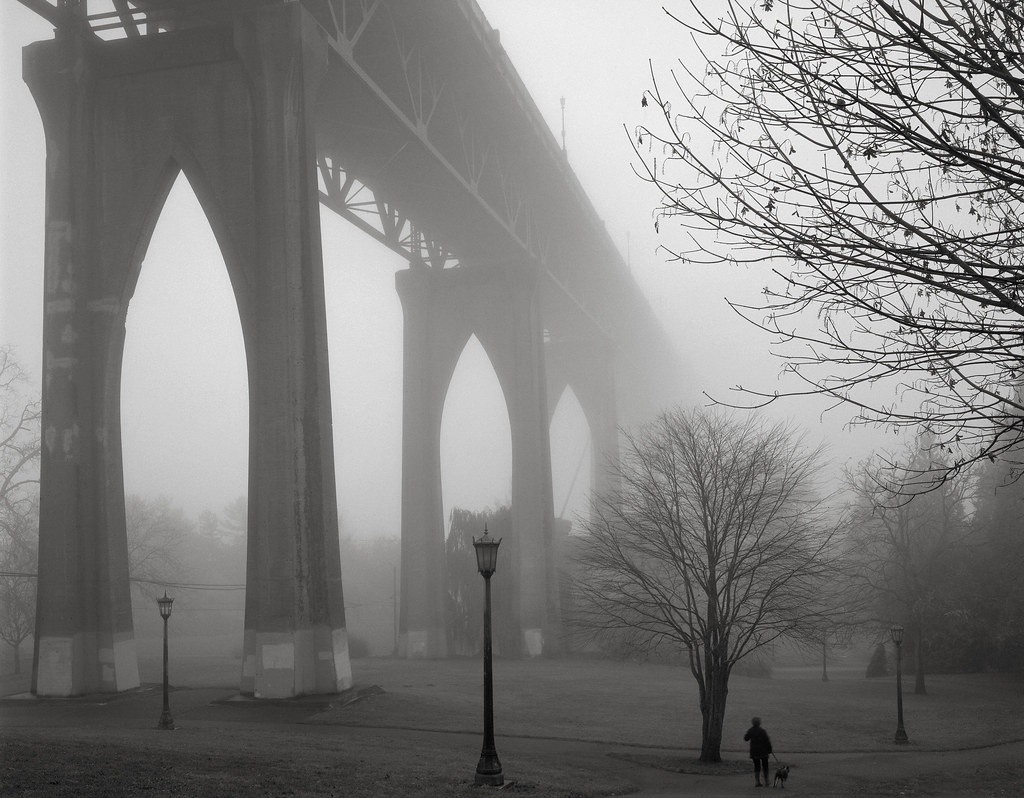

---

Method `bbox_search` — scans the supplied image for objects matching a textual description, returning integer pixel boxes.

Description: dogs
[772,765,790,789]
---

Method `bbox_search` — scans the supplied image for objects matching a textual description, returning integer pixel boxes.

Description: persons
[744,716,773,787]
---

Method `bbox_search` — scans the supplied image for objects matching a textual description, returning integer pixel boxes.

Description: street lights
[470,524,507,792]
[891,626,907,742]
[914,599,926,694]
[820,618,829,683]
[154,591,176,736]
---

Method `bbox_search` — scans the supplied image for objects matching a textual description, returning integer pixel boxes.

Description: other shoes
[766,783,771,787]
[755,782,763,787]
